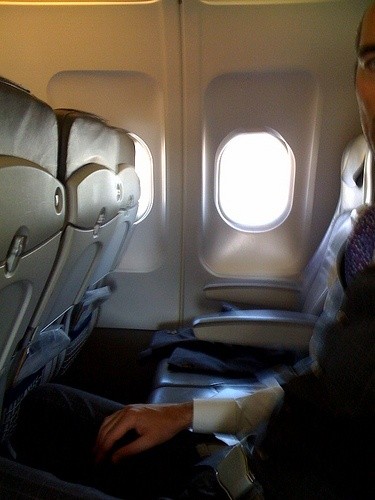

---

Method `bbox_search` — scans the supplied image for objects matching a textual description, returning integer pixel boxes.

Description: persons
[0,4,375,500]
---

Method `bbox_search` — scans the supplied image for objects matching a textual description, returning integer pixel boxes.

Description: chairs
[150,133,375,448]
[0,77,141,406]
